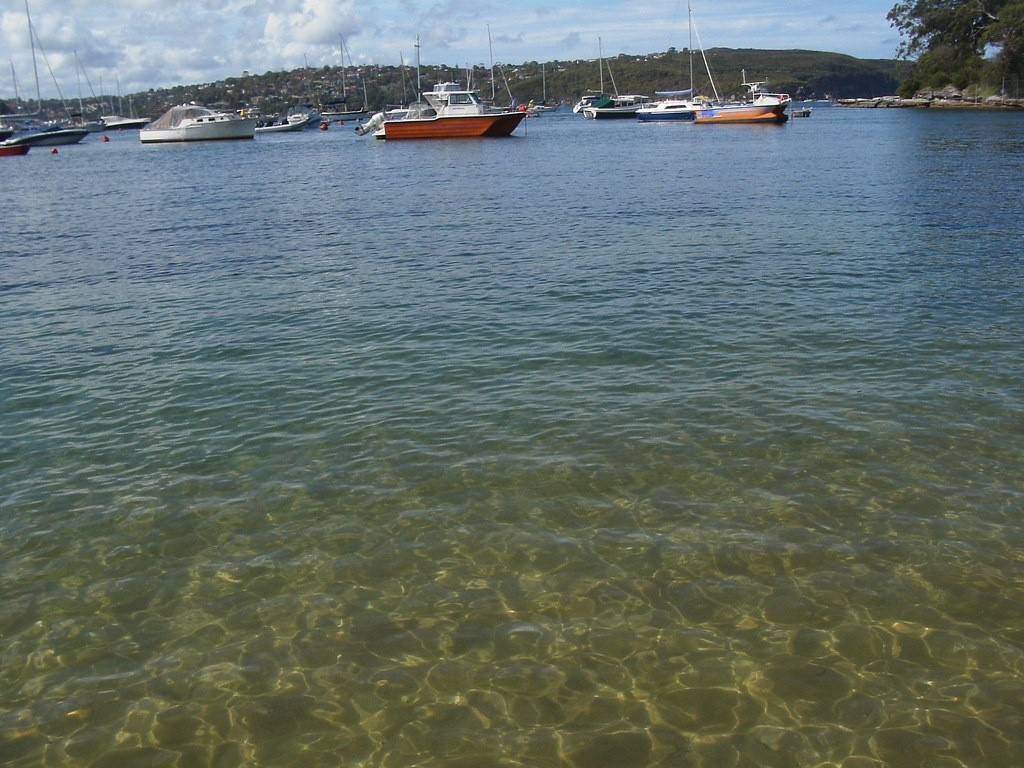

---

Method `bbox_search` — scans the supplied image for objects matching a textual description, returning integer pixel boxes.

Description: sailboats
[526,62,559,112]
[354,21,514,114]
[318,33,366,121]
[634,0,719,121]
[574,36,650,119]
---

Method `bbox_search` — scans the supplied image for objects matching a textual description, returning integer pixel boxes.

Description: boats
[255,112,323,132]
[353,82,528,140]
[692,93,791,123]
[792,107,811,117]
[140,100,254,144]
[0,0,153,156]
